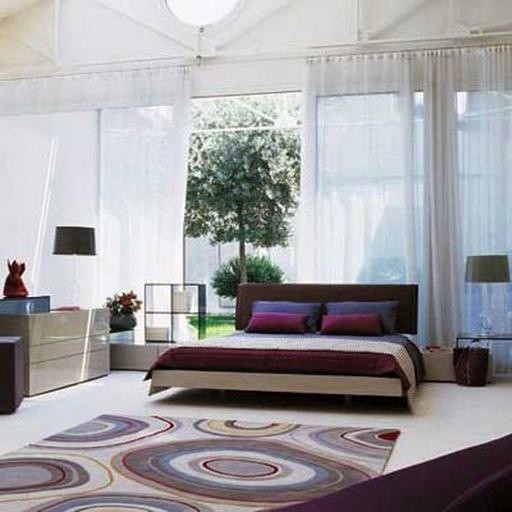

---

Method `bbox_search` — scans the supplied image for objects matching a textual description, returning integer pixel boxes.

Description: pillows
[244,300,401,337]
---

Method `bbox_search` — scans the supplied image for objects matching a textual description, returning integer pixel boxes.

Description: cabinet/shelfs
[0,308,110,397]
[144,283,205,343]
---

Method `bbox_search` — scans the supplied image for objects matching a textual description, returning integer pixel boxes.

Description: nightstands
[420,344,457,382]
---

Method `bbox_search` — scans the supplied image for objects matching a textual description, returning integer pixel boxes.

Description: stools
[0,336,24,414]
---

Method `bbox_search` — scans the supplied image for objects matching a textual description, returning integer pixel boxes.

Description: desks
[456,337,512,349]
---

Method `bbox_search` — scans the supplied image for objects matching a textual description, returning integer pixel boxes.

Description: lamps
[464,254,510,337]
[54,226,96,305]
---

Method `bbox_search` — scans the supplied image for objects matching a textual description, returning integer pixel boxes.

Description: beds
[152,283,418,405]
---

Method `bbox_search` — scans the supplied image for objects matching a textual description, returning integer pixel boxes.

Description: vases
[110,314,136,332]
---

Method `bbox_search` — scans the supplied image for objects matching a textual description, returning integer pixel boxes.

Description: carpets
[0,414,400,512]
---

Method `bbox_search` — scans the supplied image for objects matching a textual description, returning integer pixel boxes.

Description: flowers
[107,289,142,316]
